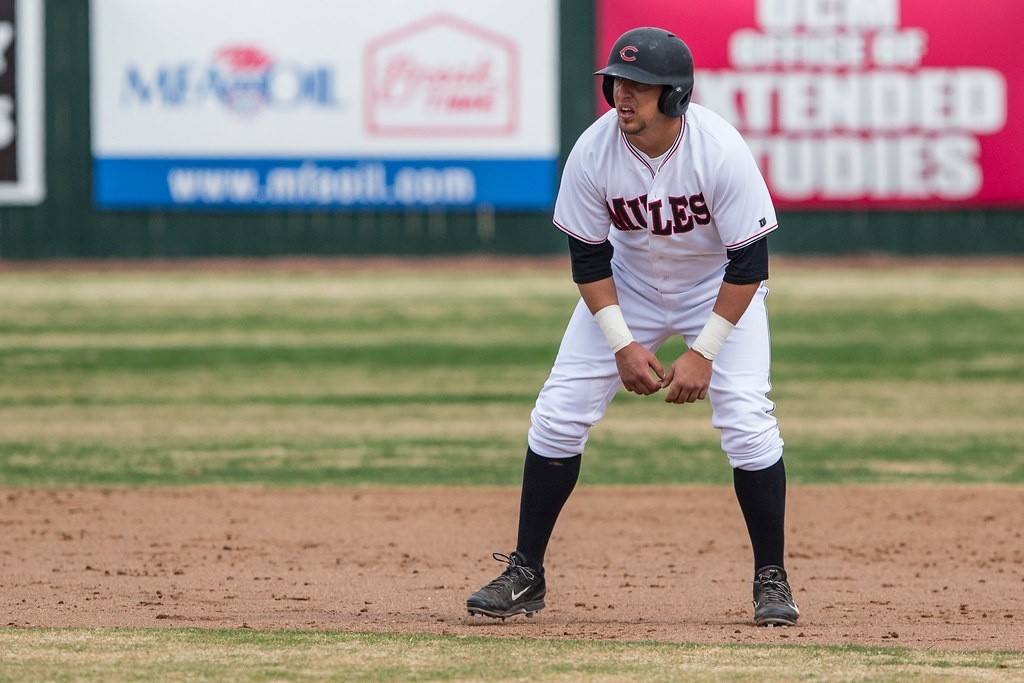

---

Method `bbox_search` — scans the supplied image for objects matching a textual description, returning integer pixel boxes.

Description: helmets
[593,27,694,117]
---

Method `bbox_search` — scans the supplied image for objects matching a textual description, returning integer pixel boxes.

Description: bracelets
[593,304,636,354]
[688,311,735,360]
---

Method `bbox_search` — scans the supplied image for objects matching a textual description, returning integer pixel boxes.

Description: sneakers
[467,552,547,616]
[754,566,800,626]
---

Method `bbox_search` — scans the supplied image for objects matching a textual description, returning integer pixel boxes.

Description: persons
[466,25,801,630]
